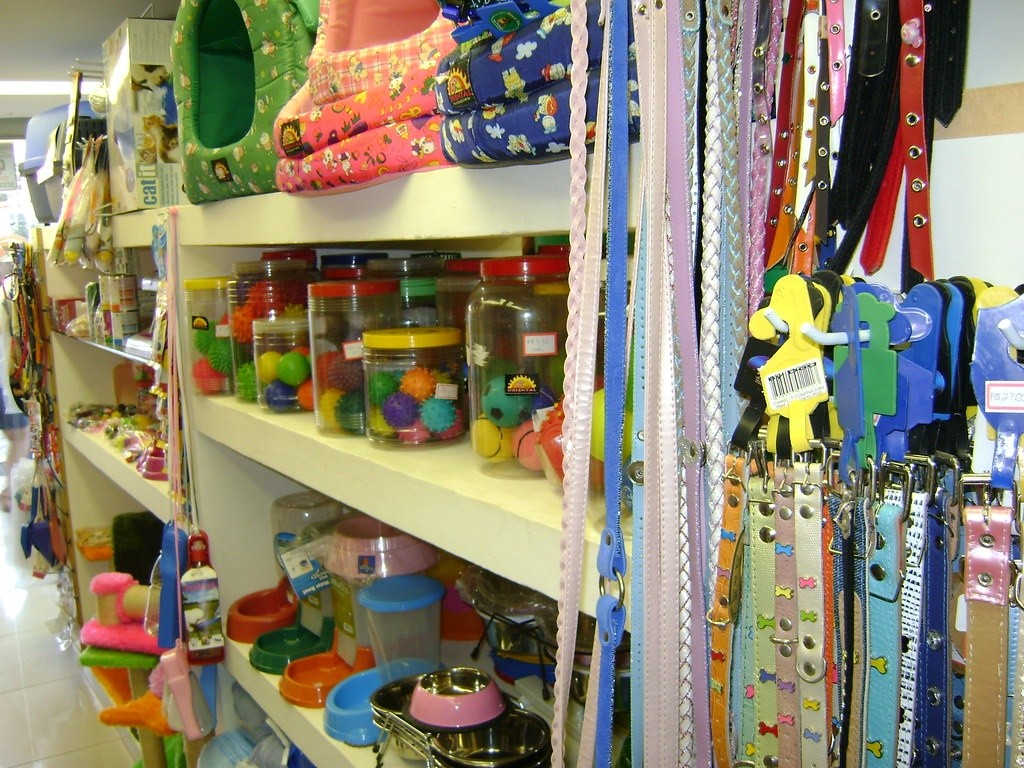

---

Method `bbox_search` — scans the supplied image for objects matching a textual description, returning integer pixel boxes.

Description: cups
[355,575,445,683]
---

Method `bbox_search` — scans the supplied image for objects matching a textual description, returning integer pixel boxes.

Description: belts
[548,1,1024,767]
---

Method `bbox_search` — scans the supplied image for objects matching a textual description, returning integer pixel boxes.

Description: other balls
[188,274,634,512]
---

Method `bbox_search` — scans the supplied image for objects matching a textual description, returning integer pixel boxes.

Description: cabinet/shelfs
[0,0,1024,766]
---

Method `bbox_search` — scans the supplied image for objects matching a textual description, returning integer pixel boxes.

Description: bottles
[183,248,639,514]
